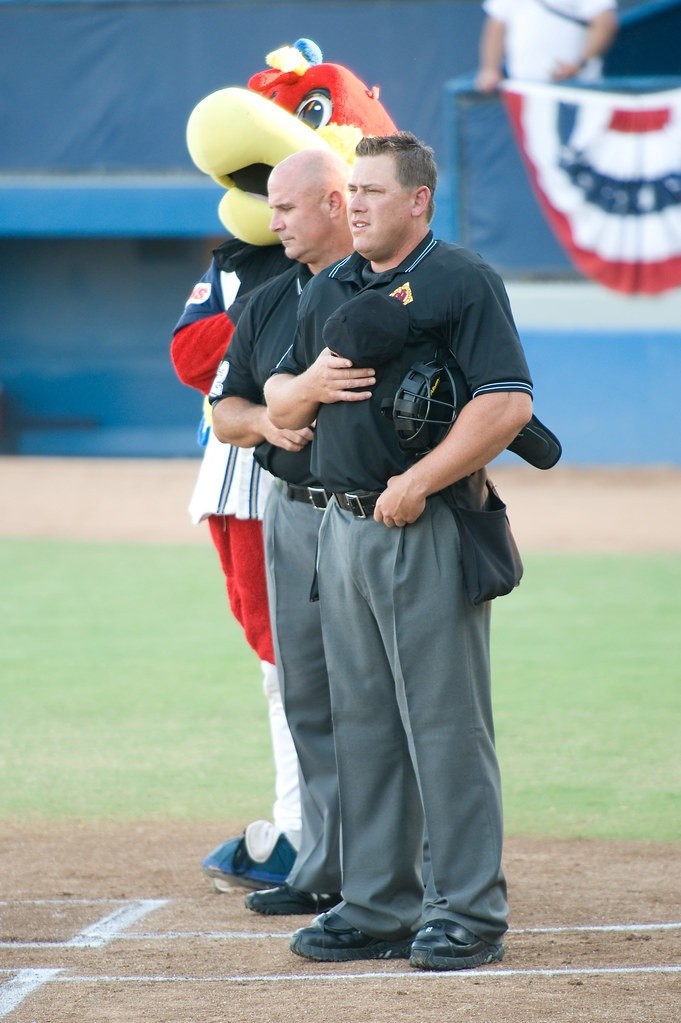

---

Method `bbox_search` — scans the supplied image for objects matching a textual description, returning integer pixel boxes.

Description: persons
[208,131,533,970]
[472,0,620,94]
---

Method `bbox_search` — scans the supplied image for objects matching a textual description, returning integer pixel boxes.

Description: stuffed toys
[169,35,403,895]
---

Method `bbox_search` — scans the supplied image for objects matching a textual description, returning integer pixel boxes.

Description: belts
[273,476,332,509]
[335,490,383,518]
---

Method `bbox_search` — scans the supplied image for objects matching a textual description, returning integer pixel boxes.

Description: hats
[322,290,410,370]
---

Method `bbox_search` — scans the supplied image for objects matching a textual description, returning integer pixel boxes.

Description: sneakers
[289,910,424,962]
[245,881,344,916]
[409,919,505,971]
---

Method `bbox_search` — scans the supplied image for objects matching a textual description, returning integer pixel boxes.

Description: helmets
[373,341,469,470]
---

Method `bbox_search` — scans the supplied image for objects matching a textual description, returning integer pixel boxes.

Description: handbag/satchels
[442,475,524,607]
